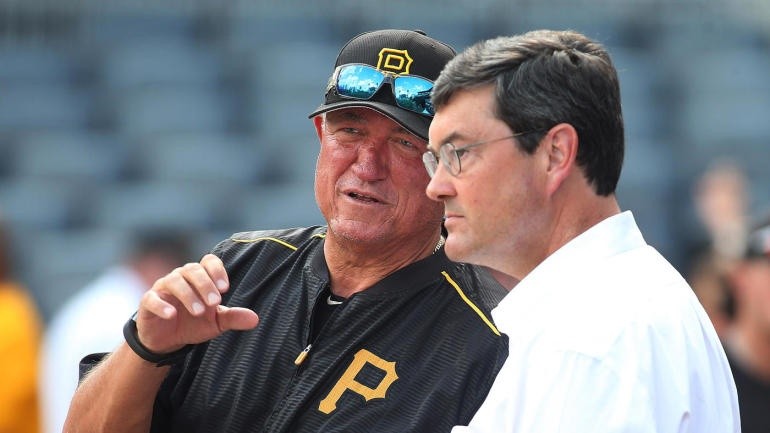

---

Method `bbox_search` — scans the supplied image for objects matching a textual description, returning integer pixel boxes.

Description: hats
[308,28,458,141]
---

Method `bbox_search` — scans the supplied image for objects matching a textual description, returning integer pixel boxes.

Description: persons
[1,227,42,433]
[42,221,193,433]
[681,160,770,433]
[61,26,509,433]
[421,27,743,433]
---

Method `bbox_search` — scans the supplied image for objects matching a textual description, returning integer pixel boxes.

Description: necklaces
[432,235,447,254]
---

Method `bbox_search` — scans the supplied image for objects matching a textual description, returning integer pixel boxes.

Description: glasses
[324,62,438,118]
[423,125,549,179]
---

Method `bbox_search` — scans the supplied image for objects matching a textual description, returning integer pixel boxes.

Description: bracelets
[123,310,195,367]
